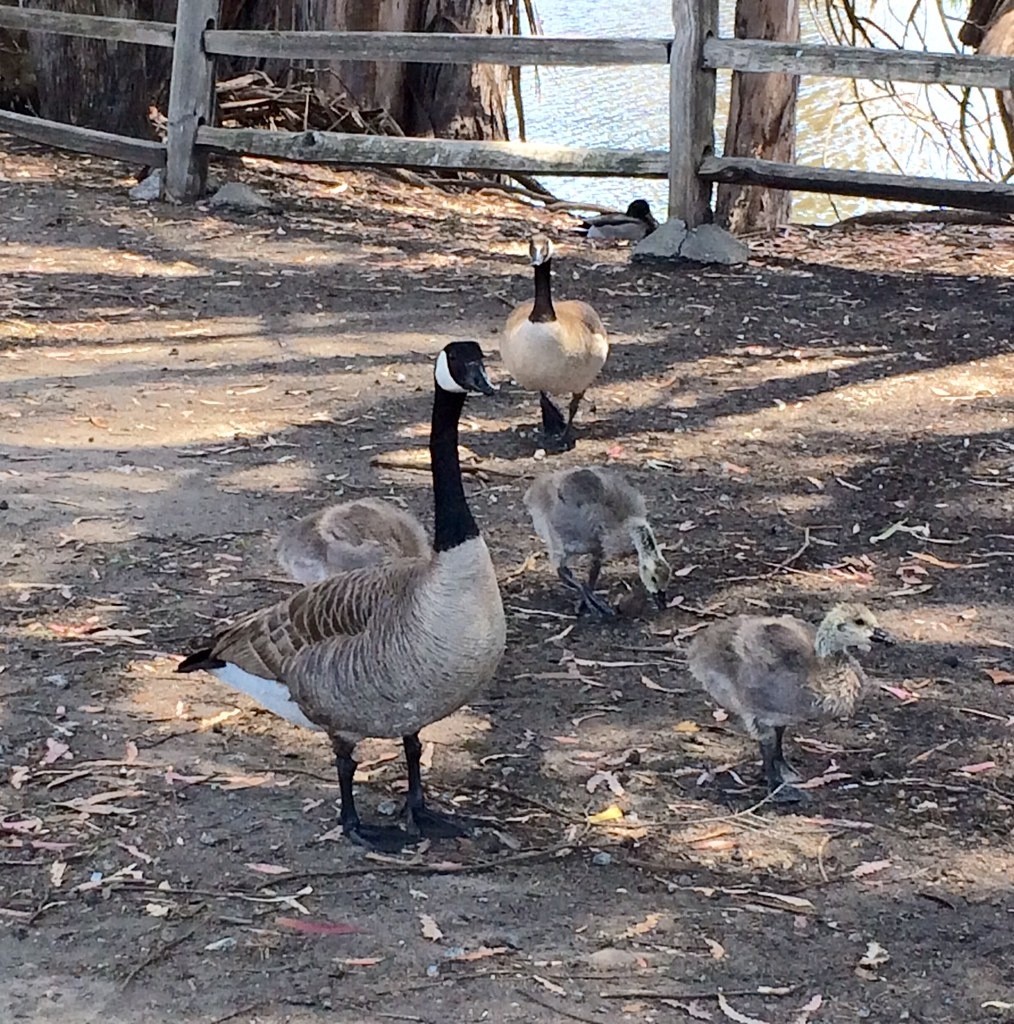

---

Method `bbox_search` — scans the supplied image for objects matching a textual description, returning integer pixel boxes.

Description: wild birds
[498,233,611,453]
[173,339,499,853]
[525,460,672,627]
[686,602,899,787]
